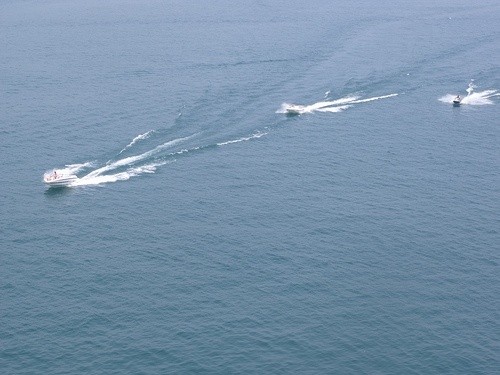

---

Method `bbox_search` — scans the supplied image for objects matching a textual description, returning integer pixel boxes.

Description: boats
[286,104,305,112]
[453,95,463,103]
[43,172,77,187]
